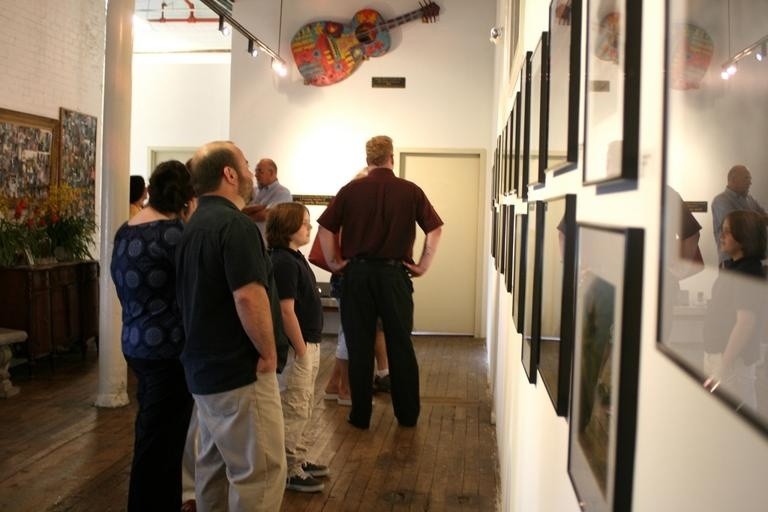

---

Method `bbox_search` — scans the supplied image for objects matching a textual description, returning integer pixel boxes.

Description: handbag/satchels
[309,196,340,272]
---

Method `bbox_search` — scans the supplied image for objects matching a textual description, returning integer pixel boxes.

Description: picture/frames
[568,222,644,512]
[59,107,98,232]
[0,108,60,223]
[492,0,581,416]
[582,0,642,186]
[656,0,768,440]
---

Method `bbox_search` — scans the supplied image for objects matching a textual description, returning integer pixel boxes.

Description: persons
[111,141,330,512]
[316,136,445,430]
[701,166,768,410]
[558,138,704,435]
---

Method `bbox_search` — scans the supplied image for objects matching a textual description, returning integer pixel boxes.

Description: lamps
[200,0,286,76]
[721,0,768,81]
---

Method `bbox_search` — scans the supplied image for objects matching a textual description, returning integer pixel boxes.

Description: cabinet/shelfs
[0,260,99,379]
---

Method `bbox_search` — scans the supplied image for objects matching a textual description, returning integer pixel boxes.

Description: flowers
[0,183,95,265]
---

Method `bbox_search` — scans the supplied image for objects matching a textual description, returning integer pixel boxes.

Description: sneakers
[302,461,329,477]
[286,468,324,493]
[375,374,391,393]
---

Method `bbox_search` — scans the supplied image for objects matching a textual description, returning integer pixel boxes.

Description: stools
[0,328,29,399]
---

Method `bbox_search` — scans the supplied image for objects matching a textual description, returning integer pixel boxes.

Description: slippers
[323,391,376,405]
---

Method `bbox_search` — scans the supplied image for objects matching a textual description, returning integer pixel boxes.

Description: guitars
[290,1,440,86]
